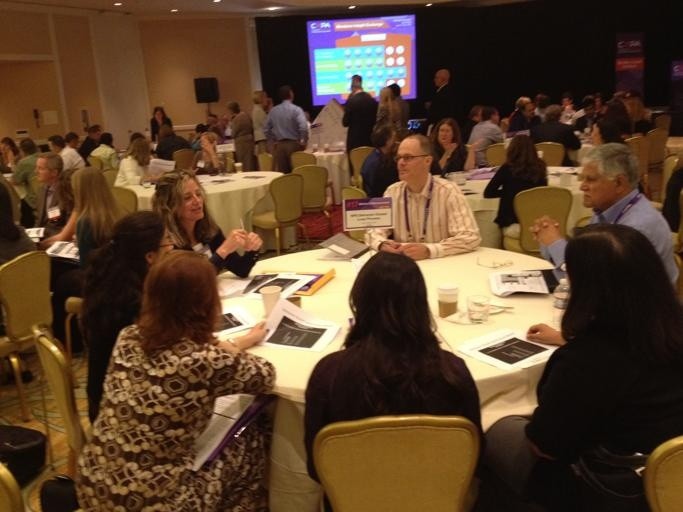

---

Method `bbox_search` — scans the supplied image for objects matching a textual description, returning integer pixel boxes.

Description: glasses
[393,153,429,162]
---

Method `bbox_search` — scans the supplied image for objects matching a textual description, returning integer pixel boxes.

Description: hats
[624,89,641,98]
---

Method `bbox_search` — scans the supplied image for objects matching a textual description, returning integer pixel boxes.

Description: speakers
[194,77,219,103]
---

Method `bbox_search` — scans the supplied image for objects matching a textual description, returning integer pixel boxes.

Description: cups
[257,285,283,317]
[437,283,459,318]
[142,177,150,189]
[466,294,490,326]
[286,296,301,308]
[559,173,572,186]
[451,171,465,186]
[232,162,242,175]
[310,141,347,154]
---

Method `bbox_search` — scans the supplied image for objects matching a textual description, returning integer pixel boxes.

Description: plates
[487,301,506,316]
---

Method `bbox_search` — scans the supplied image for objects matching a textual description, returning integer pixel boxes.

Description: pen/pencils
[240,219,244,229]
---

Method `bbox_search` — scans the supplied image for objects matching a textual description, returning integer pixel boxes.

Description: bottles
[549,278,569,331]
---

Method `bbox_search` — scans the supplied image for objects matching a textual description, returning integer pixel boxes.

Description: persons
[0,65,682,511]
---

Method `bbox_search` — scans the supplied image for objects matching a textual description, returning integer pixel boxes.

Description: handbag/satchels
[562,444,650,501]
